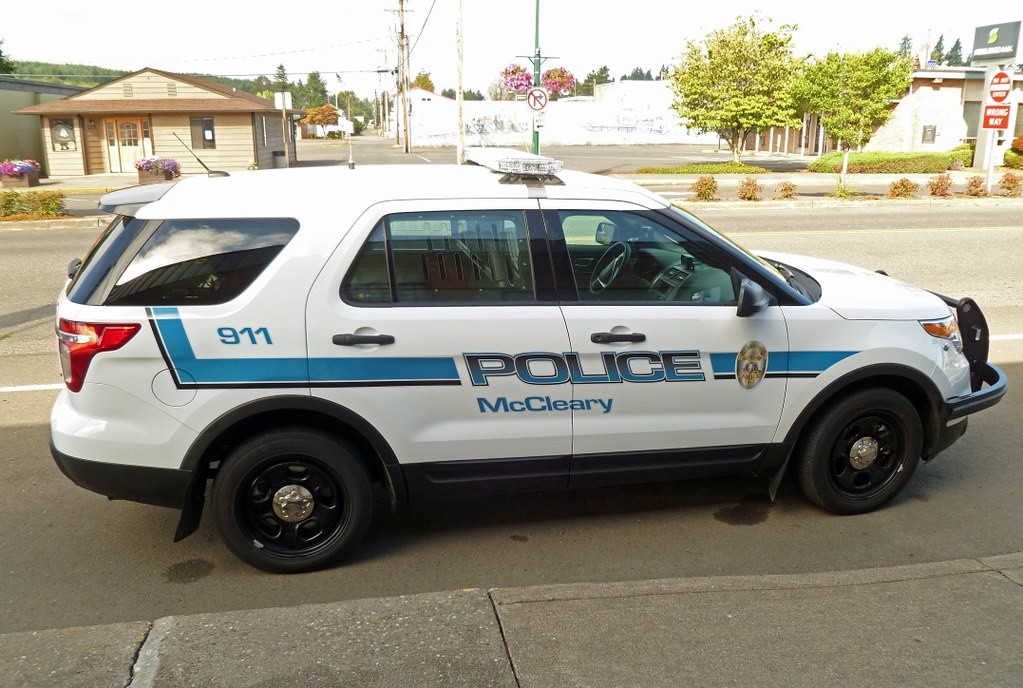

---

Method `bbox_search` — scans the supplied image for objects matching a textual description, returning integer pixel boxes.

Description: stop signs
[988,72,1011,103]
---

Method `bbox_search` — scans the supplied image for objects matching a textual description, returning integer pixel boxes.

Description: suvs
[45,146,1010,574]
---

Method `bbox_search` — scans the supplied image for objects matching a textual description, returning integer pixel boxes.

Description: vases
[138,169,179,184]
[2,171,40,188]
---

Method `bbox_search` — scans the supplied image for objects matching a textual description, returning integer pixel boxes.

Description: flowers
[0,156,39,181]
[540,66,577,96]
[499,63,532,93]
[134,157,179,174]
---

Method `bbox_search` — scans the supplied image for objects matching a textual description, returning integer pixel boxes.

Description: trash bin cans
[273,151,287,168]
